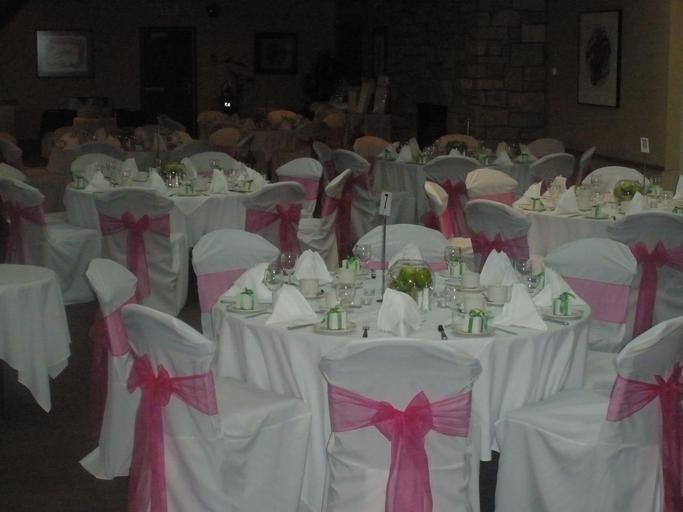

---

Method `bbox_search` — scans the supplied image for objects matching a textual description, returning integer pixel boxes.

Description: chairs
[317,337,482,510]
[464,200,543,284]
[605,211,682,339]
[120,303,312,510]
[99,189,190,315]
[1,175,102,307]
[546,237,638,353]
[1,104,682,258]
[192,230,282,341]
[494,315,682,511]
[80,258,138,480]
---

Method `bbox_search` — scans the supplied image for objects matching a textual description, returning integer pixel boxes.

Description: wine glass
[224,240,583,338]
[418,141,531,168]
[71,158,254,198]
[518,174,683,219]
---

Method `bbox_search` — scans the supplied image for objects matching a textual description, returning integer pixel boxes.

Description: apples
[615,180,640,195]
[398,267,431,292]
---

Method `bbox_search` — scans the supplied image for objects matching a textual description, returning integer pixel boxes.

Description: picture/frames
[253,31,301,79]
[576,9,624,109]
[34,28,92,81]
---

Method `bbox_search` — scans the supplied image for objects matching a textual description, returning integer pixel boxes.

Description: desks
[2,265,72,412]
[212,266,592,510]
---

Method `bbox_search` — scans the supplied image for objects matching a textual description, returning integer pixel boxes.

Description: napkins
[501,283,548,329]
[217,263,273,304]
[293,249,335,284]
[479,248,519,284]
[533,267,587,308]
[377,288,421,338]
[265,284,315,329]
